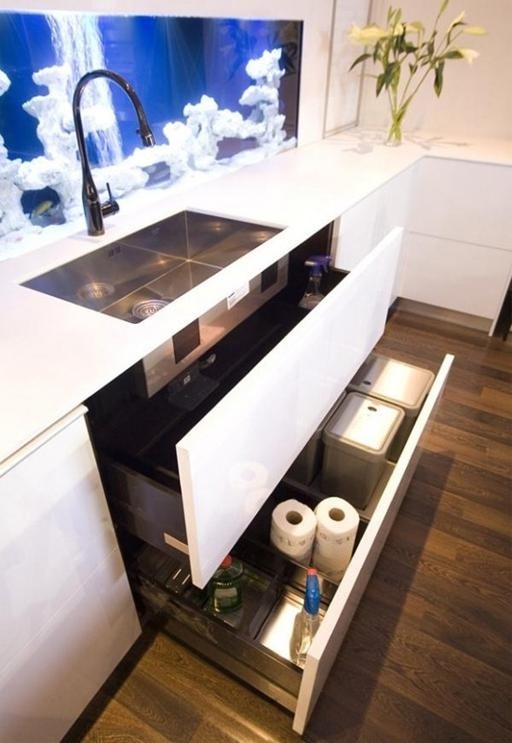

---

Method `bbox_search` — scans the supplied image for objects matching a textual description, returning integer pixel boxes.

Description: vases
[386,108,406,144]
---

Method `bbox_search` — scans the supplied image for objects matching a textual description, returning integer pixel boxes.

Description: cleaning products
[293,572,321,666]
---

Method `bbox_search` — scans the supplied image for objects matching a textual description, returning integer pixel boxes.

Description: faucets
[72,70,154,233]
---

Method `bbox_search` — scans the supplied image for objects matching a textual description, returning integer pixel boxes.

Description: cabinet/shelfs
[88,219,454,735]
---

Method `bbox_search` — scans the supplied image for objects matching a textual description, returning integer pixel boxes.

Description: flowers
[348,0,488,142]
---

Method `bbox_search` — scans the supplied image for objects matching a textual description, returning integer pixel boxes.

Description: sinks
[21,210,288,324]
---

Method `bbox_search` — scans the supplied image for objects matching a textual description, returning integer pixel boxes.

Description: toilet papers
[273,497,316,566]
[315,496,360,582]
